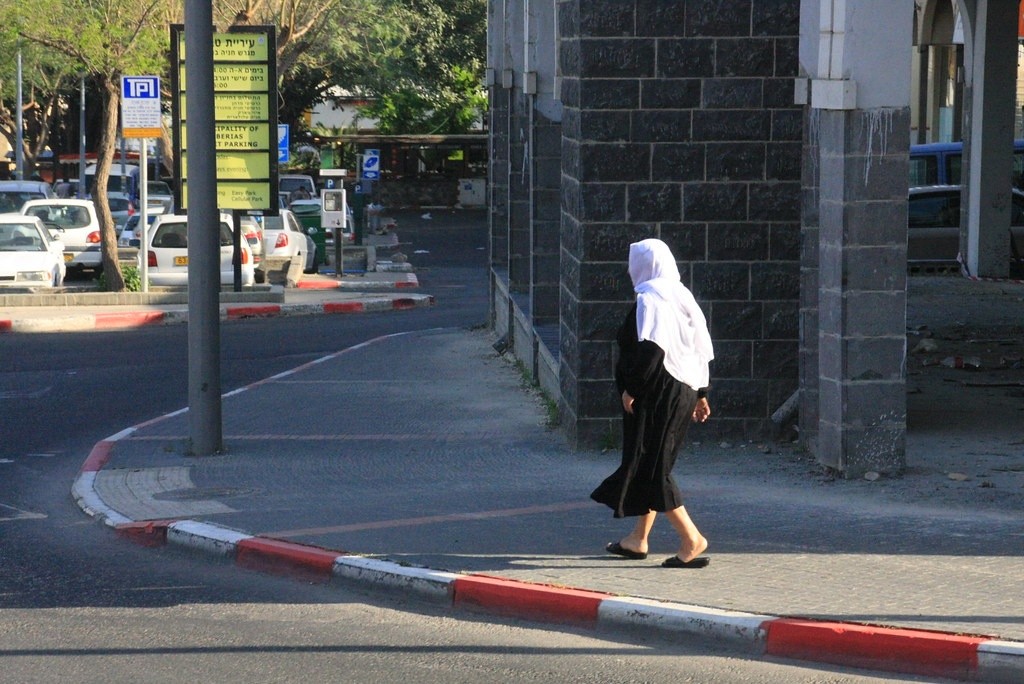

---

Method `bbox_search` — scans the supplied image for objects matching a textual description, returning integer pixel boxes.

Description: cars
[908,183,1024,272]
[0,163,356,288]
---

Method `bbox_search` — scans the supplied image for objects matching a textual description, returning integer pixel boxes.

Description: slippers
[662,557,711,568]
[606,540,647,559]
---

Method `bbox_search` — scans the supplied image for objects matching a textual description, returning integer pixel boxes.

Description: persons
[589,237,716,568]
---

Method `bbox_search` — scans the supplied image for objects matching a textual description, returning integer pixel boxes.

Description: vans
[909,139,1024,217]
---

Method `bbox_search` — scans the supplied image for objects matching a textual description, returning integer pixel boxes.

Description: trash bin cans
[291,204,326,264]
